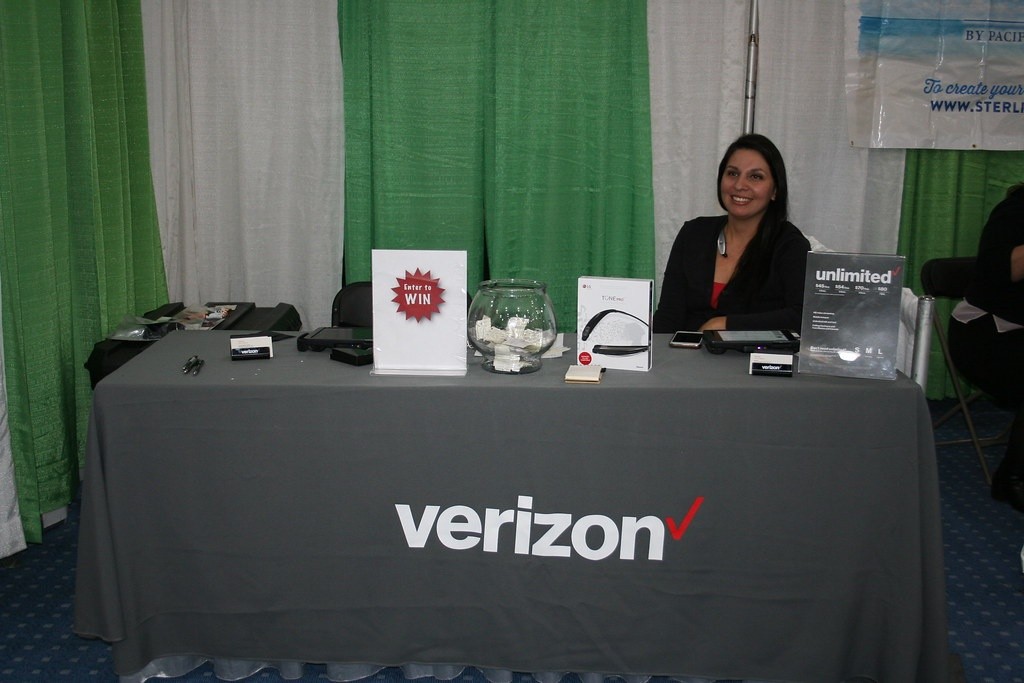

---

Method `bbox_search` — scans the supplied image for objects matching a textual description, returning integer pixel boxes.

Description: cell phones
[669,331,706,348]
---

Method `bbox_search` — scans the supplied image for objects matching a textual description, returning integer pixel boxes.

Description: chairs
[921,256,1024,486]
[331,283,473,330]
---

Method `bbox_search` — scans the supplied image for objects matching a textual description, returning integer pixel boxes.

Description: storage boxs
[576,274,655,371]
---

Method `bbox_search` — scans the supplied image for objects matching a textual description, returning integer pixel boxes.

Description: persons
[653,134,811,334]
[947,184,1024,514]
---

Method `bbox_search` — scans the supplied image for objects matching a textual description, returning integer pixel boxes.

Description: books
[798,250,905,381]
[564,364,602,384]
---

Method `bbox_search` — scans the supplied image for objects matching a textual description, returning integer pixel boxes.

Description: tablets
[704,329,801,349]
[304,327,373,350]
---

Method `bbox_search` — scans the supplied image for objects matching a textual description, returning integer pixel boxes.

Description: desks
[72,329,952,683]
